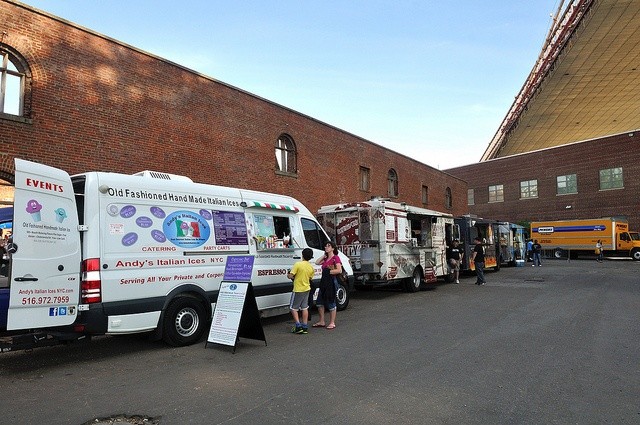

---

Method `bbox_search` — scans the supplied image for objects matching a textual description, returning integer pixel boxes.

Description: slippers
[312,323,325,327]
[327,325,336,329]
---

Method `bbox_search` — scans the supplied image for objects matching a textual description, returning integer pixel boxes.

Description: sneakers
[539,264,541,266]
[456,280,459,284]
[295,326,304,334]
[532,265,535,266]
[303,328,308,333]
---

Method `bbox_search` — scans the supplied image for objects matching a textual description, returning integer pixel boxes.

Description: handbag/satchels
[336,266,349,283]
[595,248,600,254]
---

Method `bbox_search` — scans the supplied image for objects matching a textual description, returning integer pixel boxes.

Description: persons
[448,241,464,284]
[313,241,342,328]
[473,238,487,285]
[531,239,542,267]
[287,248,313,334]
[527,238,533,261]
[600,242,603,259]
[596,240,600,261]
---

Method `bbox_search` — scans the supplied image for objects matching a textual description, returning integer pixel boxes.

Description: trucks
[531,216,640,261]
[455,214,504,276]
[499,222,528,266]
[316,197,461,292]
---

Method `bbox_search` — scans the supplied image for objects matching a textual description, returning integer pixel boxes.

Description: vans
[5,157,357,347]
